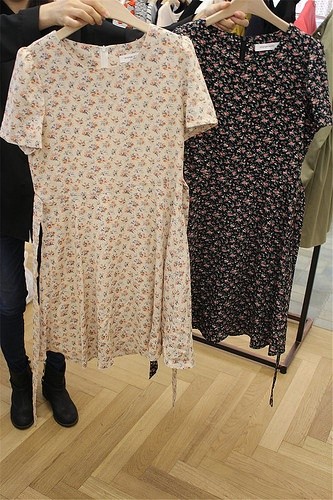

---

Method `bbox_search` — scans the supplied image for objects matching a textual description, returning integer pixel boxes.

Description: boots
[1,312,78,430]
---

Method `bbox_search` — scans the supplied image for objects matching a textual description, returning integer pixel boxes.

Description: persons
[0,0,249,429]
